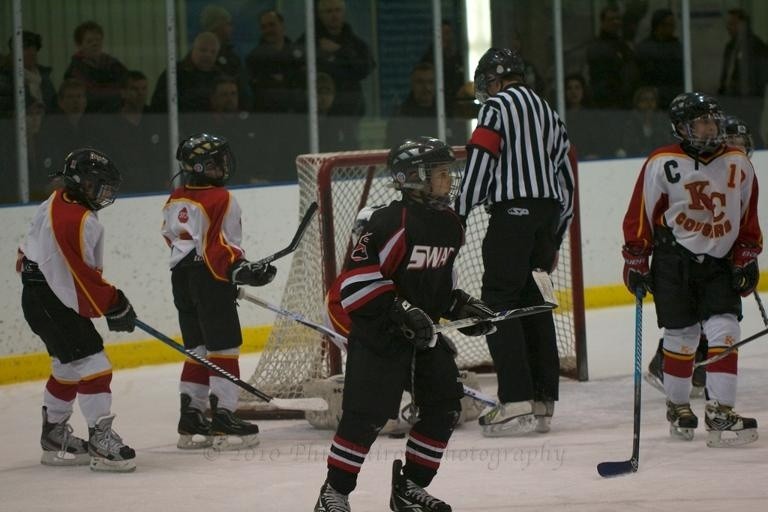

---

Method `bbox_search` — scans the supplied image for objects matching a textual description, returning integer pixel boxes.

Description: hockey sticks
[254,202,319,264]
[597,276,643,478]
[403,268,559,339]
[135,317,330,410]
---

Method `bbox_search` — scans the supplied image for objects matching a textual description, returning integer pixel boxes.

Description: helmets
[176,131,236,185]
[475,47,525,104]
[726,116,754,158]
[387,135,463,212]
[61,148,121,210]
[669,93,727,148]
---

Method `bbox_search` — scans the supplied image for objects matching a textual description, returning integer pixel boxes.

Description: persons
[621,93,763,431]
[17,147,136,461]
[314,136,498,512]
[648,113,754,388]
[161,133,276,435]
[455,45,574,424]
[0,2,768,197]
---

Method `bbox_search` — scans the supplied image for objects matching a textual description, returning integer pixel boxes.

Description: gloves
[732,242,759,297]
[227,259,276,287]
[390,295,437,348]
[621,240,653,297]
[442,290,497,336]
[103,288,137,333]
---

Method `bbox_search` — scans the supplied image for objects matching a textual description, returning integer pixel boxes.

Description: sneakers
[479,398,534,426]
[534,400,554,415]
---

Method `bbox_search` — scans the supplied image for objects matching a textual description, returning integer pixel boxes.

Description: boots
[390,459,452,512]
[649,339,664,382]
[705,388,757,432]
[210,395,259,436]
[40,406,88,453]
[692,350,706,386]
[665,397,697,427]
[177,392,210,435]
[319,478,350,511]
[87,414,135,460]
